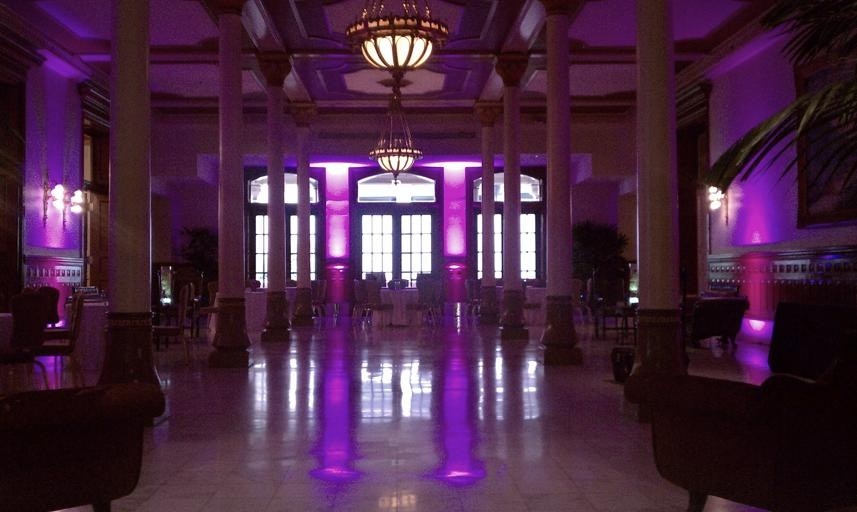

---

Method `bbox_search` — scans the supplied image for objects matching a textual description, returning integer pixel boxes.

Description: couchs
[691,297,751,345]
[647,343,857,512]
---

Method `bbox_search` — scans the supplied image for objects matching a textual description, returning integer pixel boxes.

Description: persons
[586,269,605,341]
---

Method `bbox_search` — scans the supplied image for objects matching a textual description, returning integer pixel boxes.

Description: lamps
[345,0,449,81]
[367,79,423,185]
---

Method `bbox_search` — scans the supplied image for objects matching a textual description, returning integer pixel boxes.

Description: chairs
[464,276,548,327]
[247,279,331,334]
[571,273,639,357]
[1,264,212,397]
[353,270,447,329]
